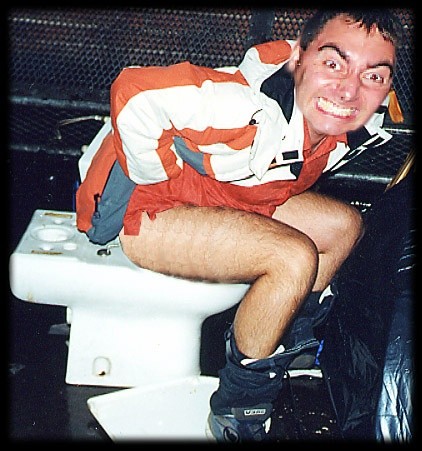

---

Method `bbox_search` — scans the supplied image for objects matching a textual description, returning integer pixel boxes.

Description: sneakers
[205,405,283,446]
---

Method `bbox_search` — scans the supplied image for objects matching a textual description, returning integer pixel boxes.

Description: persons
[74,10,403,441]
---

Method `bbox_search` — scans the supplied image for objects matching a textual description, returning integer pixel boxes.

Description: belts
[225,296,338,380]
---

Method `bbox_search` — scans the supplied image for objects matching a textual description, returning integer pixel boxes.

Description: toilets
[8,209,252,389]
